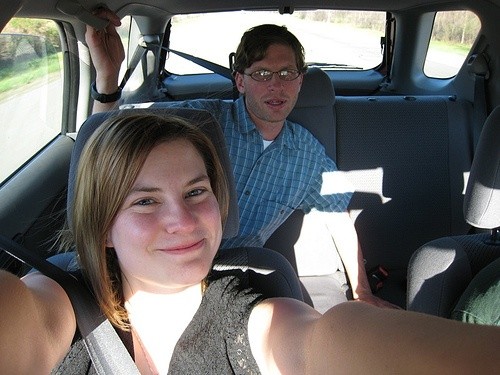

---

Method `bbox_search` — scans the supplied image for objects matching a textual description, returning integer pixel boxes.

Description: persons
[85,6,405,309]
[0,114,500,375]
[453,259,500,326]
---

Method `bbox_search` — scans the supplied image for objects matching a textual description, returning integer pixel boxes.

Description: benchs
[121,68,489,309]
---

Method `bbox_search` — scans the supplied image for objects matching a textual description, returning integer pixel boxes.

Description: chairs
[29,109,304,345]
[407,106,500,318]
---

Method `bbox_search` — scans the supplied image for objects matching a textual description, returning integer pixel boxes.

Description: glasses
[242,69,303,82]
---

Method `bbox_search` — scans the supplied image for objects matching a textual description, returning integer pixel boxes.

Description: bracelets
[92,82,121,102]
